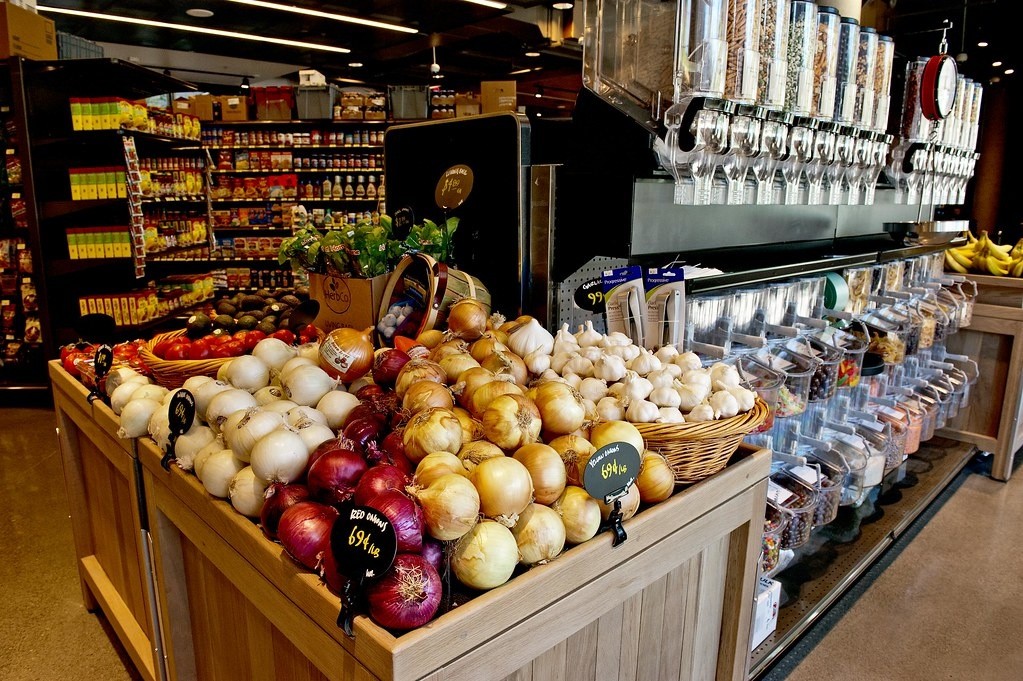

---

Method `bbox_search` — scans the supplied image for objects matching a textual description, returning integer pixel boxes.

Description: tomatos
[60,325,316,395]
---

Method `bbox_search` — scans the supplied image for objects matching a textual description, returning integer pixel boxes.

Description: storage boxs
[308,272,393,345]
[211,237,282,258]
[252,86,293,121]
[295,85,344,119]
[65,226,132,259]
[78,288,159,326]
[174,112,201,141]
[219,151,292,169]
[170,93,213,121]
[365,93,386,106]
[167,274,214,304]
[157,169,203,197]
[219,174,298,199]
[70,97,150,133]
[221,95,249,122]
[342,91,363,106]
[388,84,430,119]
[481,80,517,114]
[343,107,363,120]
[456,105,479,118]
[364,108,387,119]
[432,94,456,105]
[69,166,128,201]
[212,203,298,227]
[55,30,104,60]
[433,112,455,118]
[456,93,480,105]
[239,268,250,288]
[299,70,326,85]
[226,267,238,289]
[157,217,207,245]
[0,2,58,60]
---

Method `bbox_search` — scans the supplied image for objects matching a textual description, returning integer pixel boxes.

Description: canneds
[293,128,385,168]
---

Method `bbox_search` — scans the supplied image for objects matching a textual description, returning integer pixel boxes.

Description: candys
[749,357,860,573]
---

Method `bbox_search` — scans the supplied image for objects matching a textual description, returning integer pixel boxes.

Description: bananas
[943,230,1023,278]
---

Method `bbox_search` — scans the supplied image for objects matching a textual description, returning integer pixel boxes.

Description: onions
[105,325,442,628]
[395,298,677,590]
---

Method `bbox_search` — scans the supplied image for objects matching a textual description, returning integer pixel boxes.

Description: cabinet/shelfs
[46,358,169,681]
[25,57,217,359]
[201,119,445,292]
[138,436,774,681]
[933,272,1023,483]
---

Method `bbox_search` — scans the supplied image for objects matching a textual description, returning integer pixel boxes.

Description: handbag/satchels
[306,263,395,343]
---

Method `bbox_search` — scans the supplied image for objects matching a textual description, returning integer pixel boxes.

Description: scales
[882,18,970,246]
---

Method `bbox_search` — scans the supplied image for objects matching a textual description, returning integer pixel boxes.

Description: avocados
[184,287,310,333]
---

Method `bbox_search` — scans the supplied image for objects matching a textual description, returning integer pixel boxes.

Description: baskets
[589,383,771,491]
[139,323,325,390]
[375,249,492,353]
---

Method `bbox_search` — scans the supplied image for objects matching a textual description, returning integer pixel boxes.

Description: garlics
[377,303,413,339]
[512,316,758,423]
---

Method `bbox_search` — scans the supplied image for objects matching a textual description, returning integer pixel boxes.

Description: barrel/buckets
[376,252,491,354]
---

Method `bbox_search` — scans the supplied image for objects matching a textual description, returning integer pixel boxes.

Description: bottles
[637,0,983,153]
[138,128,386,227]
[431,90,456,113]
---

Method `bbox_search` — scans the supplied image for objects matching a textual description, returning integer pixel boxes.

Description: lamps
[240,77,250,89]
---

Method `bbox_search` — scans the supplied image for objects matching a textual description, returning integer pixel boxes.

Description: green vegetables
[278,214,461,277]
